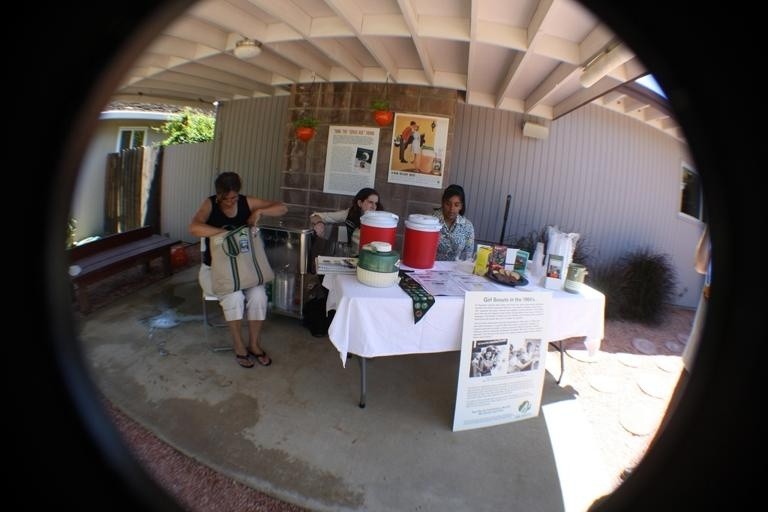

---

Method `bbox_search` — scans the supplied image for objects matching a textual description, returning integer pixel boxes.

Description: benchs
[65,223,182,317]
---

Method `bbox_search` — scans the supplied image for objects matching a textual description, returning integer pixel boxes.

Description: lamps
[233,38,263,59]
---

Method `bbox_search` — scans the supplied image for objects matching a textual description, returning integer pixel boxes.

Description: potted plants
[294,117,321,141]
[371,98,393,126]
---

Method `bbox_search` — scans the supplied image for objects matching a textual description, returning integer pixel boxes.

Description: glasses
[219,196,240,203]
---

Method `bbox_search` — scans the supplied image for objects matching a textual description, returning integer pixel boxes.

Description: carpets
[75,243,690,511]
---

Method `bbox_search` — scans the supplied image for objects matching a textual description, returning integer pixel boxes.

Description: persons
[409,125,421,163]
[508,342,540,372]
[189,171,289,368]
[308,187,383,258]
[471,345,496,378]
[619,226,713,480]
[398,121,415,163]
[430,184,475,262]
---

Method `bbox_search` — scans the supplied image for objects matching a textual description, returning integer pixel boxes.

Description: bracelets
[315,220,326,226]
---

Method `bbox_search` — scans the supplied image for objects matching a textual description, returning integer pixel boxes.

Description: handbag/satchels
[209,223,274,295]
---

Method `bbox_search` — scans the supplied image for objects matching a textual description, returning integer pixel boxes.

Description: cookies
[493,267,520,282]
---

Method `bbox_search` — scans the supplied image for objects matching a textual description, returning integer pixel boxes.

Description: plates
[486,271,530,287]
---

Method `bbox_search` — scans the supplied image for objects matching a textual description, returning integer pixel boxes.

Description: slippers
[234,345,272,368]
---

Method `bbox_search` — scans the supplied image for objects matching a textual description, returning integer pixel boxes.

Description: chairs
[199,235,262,353]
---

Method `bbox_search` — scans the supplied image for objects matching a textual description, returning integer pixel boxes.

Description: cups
[420,150,435,173]
[432,158,441,175]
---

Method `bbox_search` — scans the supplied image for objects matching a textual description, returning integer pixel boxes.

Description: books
[314,255,359,276]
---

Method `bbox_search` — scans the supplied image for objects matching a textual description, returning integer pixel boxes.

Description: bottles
[531,241,545,268]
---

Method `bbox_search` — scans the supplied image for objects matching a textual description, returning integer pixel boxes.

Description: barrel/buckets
[402,213,442,268]
[273,264,295,311]
[358,211,399,252]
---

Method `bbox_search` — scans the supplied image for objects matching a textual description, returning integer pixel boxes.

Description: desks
[328,258,606,408]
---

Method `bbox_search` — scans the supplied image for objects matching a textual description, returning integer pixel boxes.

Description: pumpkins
[169,245,189,268]
